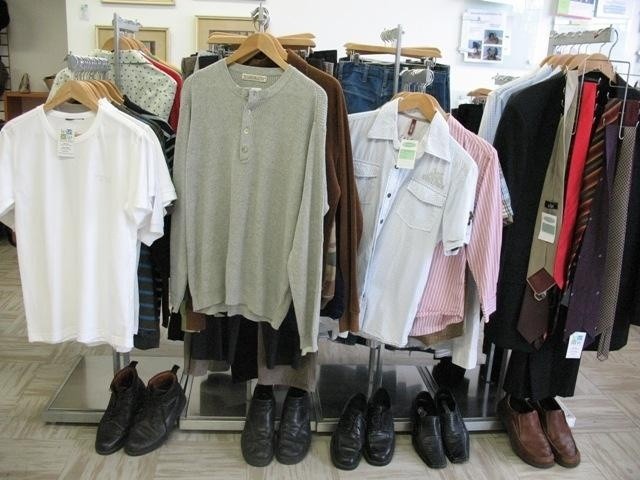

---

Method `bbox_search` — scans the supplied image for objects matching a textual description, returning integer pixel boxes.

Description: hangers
[36,11,636,141]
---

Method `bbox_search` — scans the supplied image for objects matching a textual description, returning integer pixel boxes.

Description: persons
[468,32,499,60]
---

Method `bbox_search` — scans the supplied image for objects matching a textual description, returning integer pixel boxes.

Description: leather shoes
[95,363,148,454]
[496,392,554,469]
[330,394,367,469]
[240,382,275,467]
[122,371,188,454]
[276,389,312,466]
[435,390,471,462]
[408,392,446,469]
[364,389,396,466]
[531,386,579,468]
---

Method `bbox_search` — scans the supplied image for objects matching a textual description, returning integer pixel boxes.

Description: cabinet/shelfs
[4,91,49,124]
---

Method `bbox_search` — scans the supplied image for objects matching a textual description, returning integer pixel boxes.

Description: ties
[518,70,636,360]
